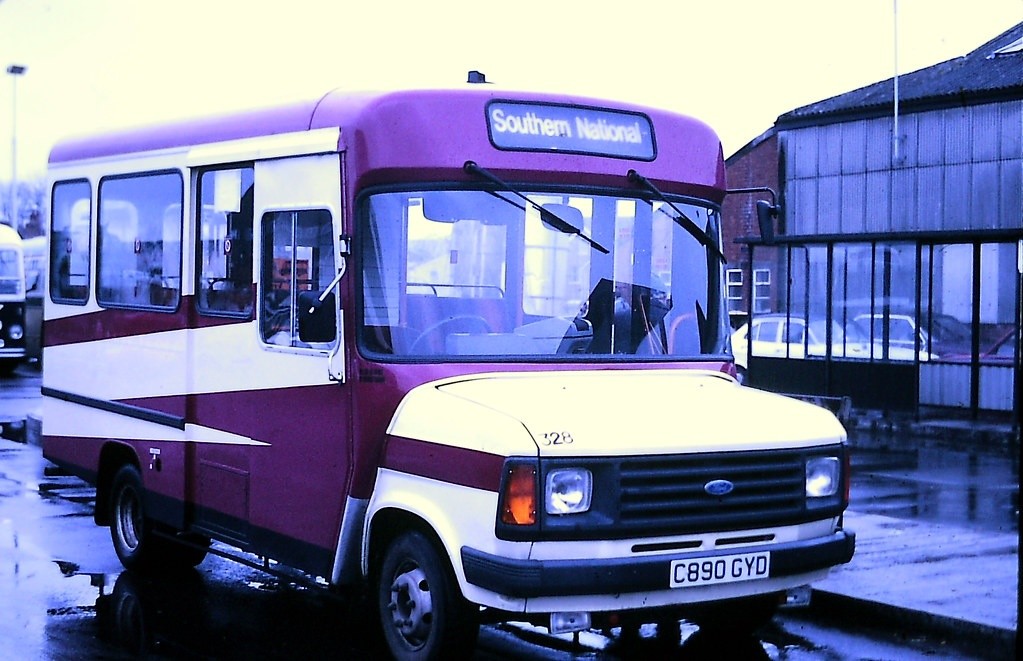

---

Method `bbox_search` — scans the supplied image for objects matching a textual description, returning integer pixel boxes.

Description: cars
[730,315,938,388]
[853,312,1014,359]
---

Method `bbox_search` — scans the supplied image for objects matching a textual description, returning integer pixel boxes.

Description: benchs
[404,284,514,354]
[58,273,252,316]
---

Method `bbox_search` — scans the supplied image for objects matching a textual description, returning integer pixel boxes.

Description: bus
[41,70,856,661]
[0,224,27,375]
